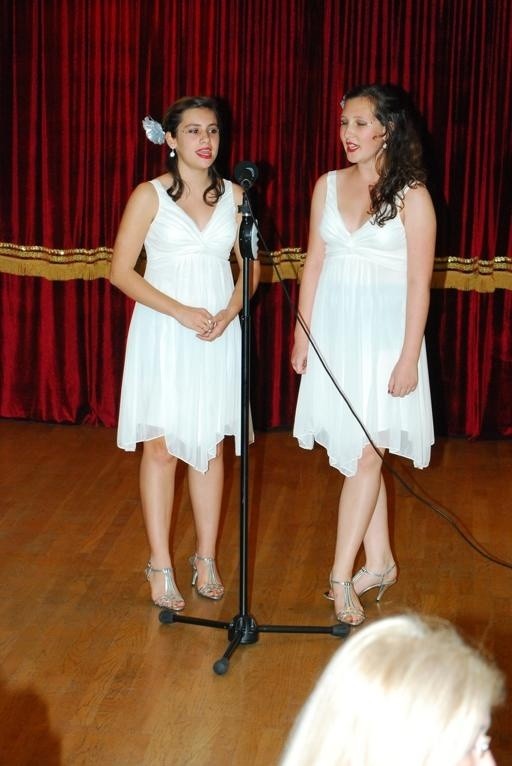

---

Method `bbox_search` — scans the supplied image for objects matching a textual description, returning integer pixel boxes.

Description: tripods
[159,190,350,673]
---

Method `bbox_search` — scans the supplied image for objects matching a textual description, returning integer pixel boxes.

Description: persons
[290,81,442,630]
[106,95,265,610]
[283,611,501,766]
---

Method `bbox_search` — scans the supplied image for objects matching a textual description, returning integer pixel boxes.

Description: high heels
[190,553,224,604]
[322,562,398,605]
[143,561,186,616]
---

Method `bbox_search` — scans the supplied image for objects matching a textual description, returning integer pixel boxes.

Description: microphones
[234,160,259,190]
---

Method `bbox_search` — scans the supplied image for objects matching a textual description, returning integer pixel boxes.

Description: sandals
[328,568,366,629]
